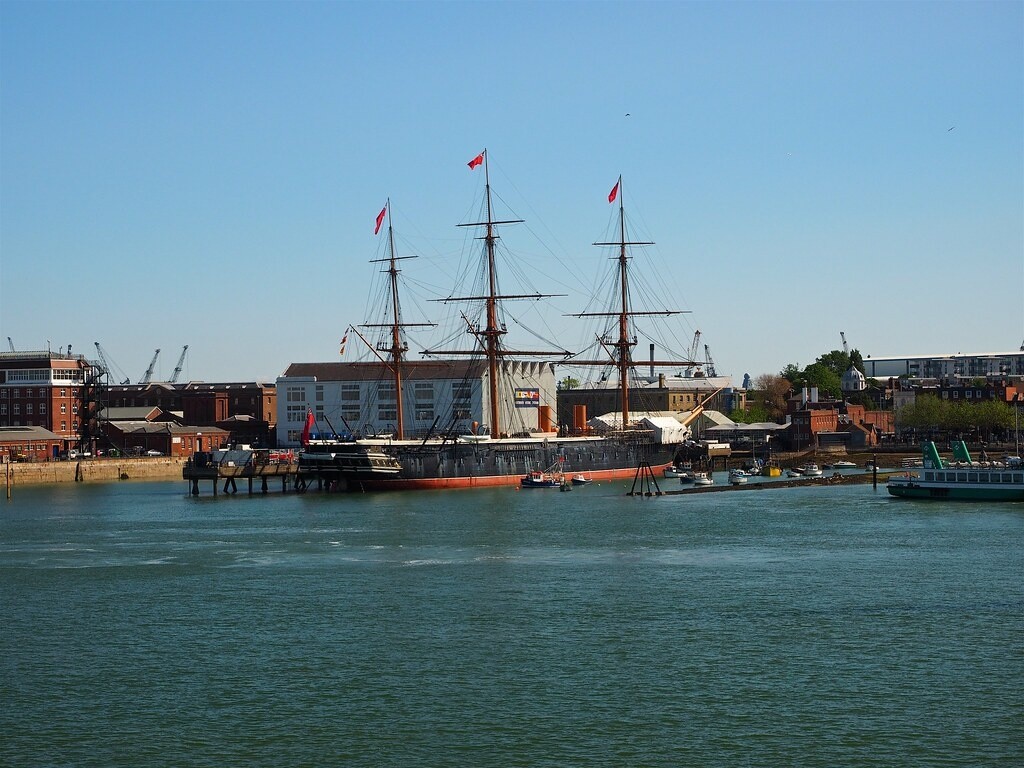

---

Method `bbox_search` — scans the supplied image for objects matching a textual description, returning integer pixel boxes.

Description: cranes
[137,349,161,384]
[169,344,190,383]
[7,336,15,352]
[94,341,130,385]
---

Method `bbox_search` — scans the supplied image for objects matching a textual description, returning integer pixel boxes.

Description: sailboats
[297,146,690,488]
[745,435,762,476]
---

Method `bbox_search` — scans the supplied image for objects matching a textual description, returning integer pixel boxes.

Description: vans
[58,450,76,461]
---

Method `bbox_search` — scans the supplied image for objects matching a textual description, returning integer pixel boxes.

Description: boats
[804,462,822,476]
[821,464,833,470]
[560,479,574,492]
[663,465,688,479]
[694,473,713,485]
[786,471,802,477]
[521,470,561,488]
[728,469,747,483]
[865,460,880,472]
[571,473,593,485]
[886,468,1024,502]
[833,461,858,469]
[901,455,1022,468]
[762,461,782,477]
[791,467,805,474]
[680,470,697,485]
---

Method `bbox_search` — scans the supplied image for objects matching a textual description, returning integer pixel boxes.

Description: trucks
[70,450,90,459]
[0,449,26,464]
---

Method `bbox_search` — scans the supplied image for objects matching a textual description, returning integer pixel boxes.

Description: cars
[144,449,164,457]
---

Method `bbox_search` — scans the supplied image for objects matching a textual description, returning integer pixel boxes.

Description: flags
[374,205,385,234]
[608,180,619,203]
[303,406,315,445]
[467,153,483,169]
